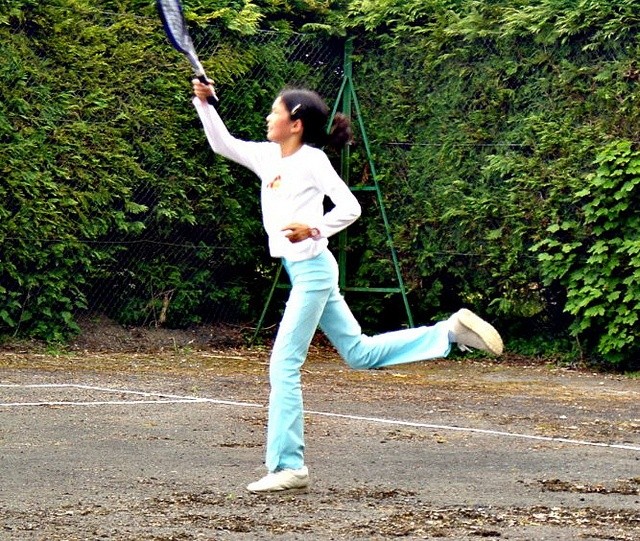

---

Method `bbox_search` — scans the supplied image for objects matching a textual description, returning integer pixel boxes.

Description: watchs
[306,222,320,245]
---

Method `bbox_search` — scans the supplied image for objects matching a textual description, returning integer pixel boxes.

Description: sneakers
[246,466,309,496]
[451,308,503,356]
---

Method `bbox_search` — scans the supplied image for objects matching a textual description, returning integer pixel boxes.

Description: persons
[191,76,505,495]
